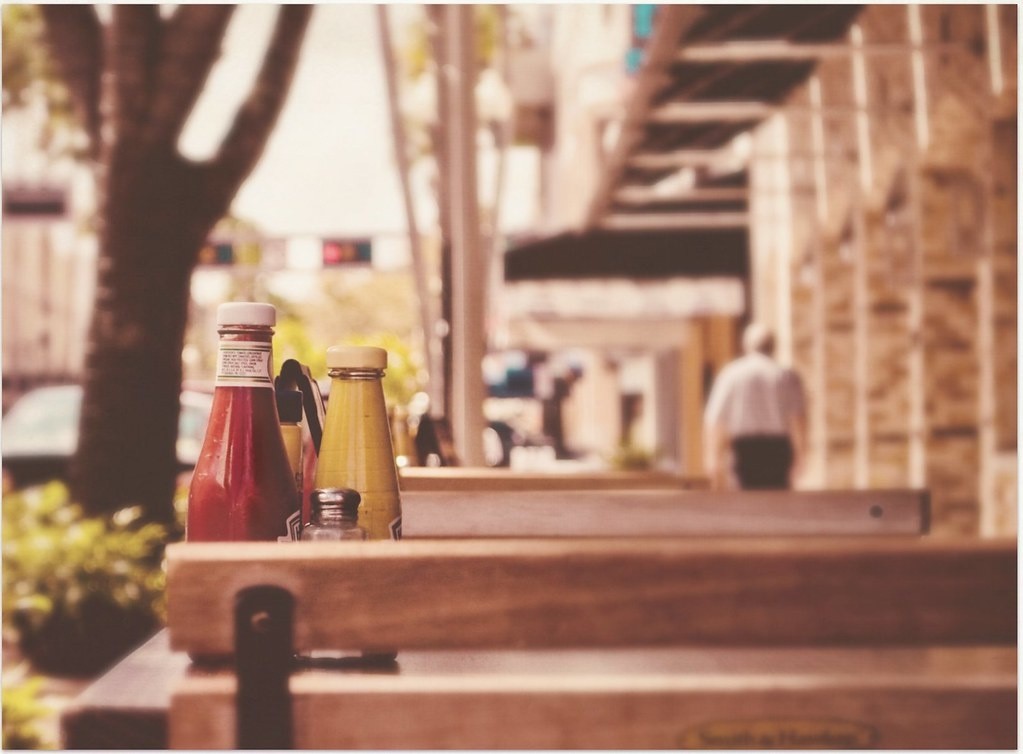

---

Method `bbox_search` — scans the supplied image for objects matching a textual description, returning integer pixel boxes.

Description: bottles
[187,302,401,540]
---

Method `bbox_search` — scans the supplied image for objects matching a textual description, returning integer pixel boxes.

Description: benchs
[162,467,1017,750]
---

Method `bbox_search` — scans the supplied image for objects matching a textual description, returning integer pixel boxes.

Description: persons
[704,323,808,490]
